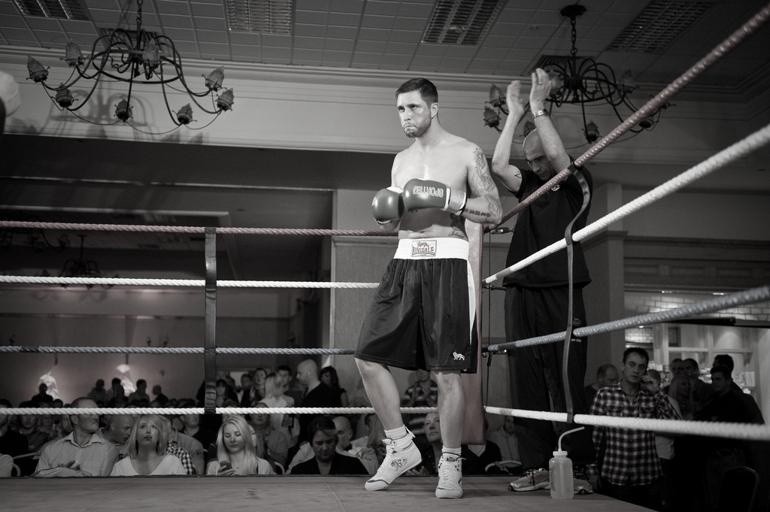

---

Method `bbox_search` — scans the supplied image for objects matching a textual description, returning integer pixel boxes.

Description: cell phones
[220,461,232,471]
[641,370,648,383]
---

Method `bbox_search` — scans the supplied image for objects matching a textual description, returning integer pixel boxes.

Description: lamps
[33,233,122,291]
[24,1,237,136]
[480,4,676,147]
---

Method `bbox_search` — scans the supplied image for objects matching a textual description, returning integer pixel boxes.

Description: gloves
[399,177,468,218]
[369,187,403,235]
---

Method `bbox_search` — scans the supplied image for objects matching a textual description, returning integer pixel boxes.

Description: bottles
[548,449,575,501]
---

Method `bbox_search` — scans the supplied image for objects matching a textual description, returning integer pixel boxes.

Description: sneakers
[435,451,466,500]
[507,466,551,493]
[573,462,594,496]
[364,426,425,492]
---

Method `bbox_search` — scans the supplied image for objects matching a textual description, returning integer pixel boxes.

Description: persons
[584,350,770,512]
[354,77,504,500]
[490,66,599,497]
[0,357,503,474]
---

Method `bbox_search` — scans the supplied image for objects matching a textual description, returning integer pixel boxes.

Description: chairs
[11,462,21,477]
[271,460,286,474]
[714,464,760,511]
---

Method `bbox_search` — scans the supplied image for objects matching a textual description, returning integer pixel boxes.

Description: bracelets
[531,108,550,121]
